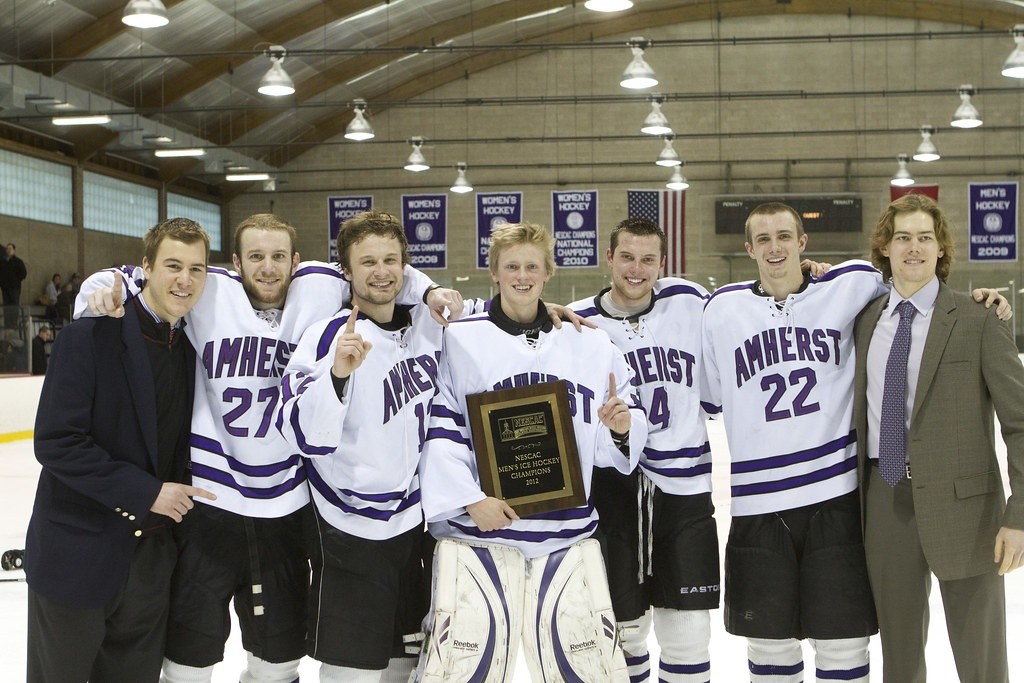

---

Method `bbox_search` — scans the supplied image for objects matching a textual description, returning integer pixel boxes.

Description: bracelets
[422,283,444,305]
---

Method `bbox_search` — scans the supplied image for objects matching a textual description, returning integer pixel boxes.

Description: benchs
[0,305,46,315]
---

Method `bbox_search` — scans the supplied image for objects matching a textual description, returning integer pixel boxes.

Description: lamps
[1001,24,1024,78]
[450,162,473,194]
[121,0,170,28]
[344,99,375,140]
[620,36,658,89]
[666,165,689,190]
[951,84,983,128]
[258,46,295,96]
[641,99,672,135]
[913,125,940,162]
[890,153,914,186]
[656,137,682,166]
[404,136,430,171]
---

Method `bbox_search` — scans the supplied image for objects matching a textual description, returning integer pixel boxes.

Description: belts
[870,458,913,478]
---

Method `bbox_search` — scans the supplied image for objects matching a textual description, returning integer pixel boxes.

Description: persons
[73,213,464,683]
[25,217,217,683]
[852,195,1024,683]
[0,244,27,326]
[565,219,831,683]
[32,326,51,374]
[276,213,598,683]
[40,272,81,326]
[409,225,648,683]
[699,203,1013,683]
[5,330,25,372]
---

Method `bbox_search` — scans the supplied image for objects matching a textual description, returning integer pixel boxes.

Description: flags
[627,190,686,279]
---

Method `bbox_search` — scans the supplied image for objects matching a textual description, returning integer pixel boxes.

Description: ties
[877,303,914,488]
